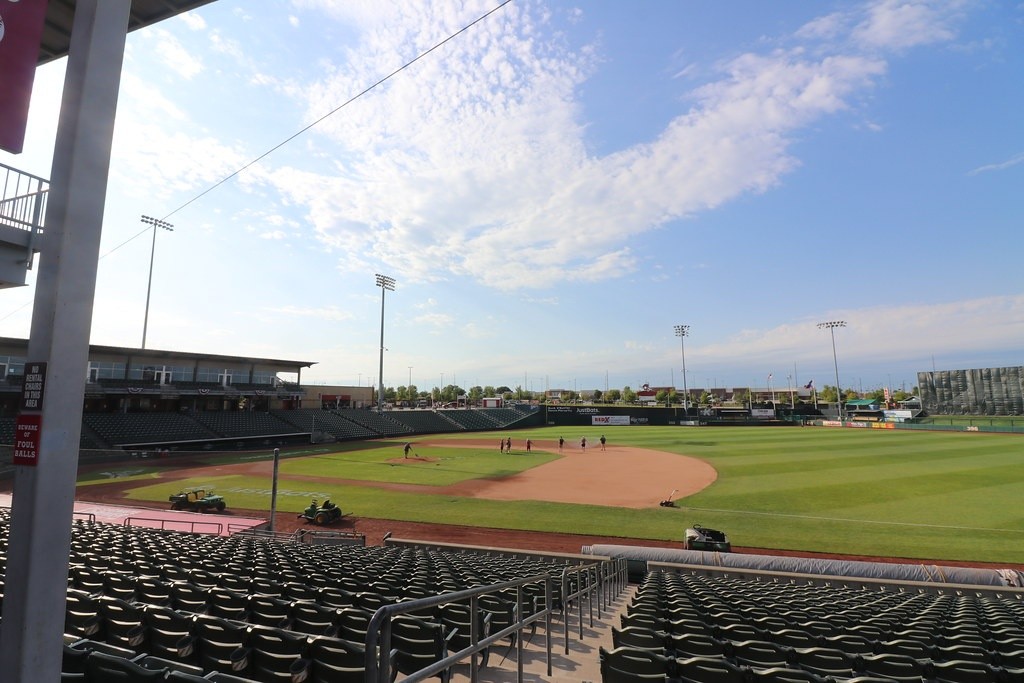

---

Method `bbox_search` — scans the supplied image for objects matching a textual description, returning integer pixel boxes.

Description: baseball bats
[410,448,419,457]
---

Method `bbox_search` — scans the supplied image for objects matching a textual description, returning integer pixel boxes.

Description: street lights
[141,214,174,349]
[375,274,396,410]
[673,325,690,411]
[816,321,847,422]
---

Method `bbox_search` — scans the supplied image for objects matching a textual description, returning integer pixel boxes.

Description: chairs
[316,500,329,509]
[0,508,1024,683]
[187,490,204,502]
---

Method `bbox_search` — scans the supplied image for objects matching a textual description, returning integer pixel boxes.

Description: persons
[558,436,564,449]
[526,438,533,452]
[600,435,606,451]
[581,436,586,452]
[404,442,411,459]
[322,500,331,509]
[500,437,512,454]
